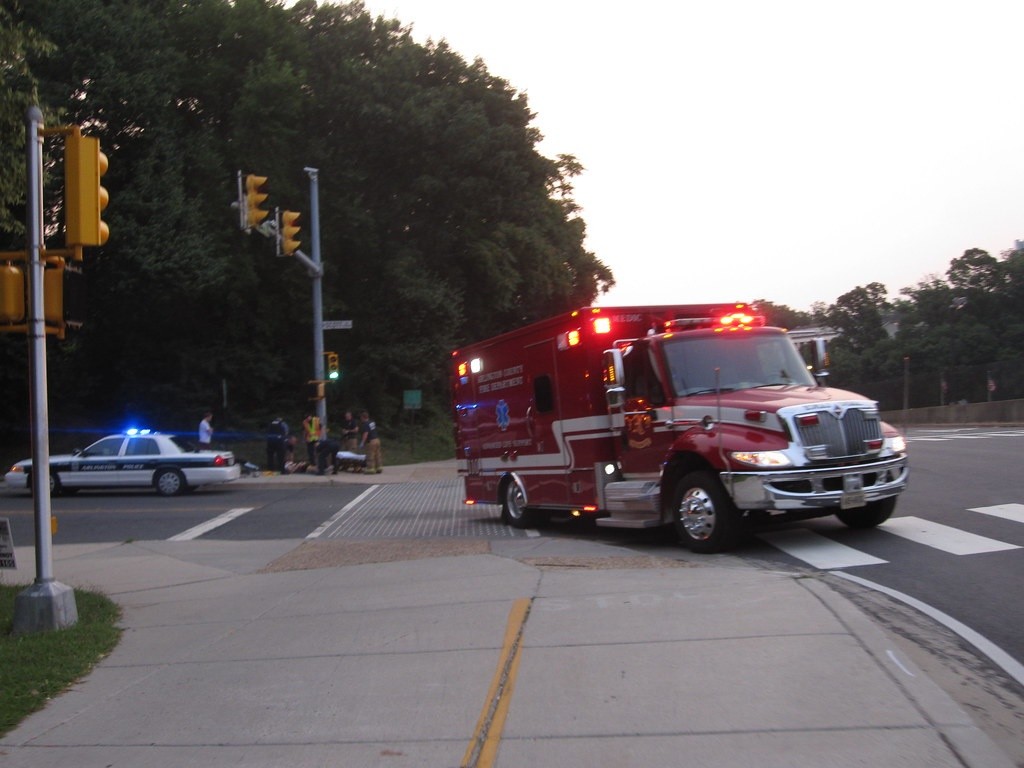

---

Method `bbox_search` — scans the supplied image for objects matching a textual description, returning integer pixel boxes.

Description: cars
[3,427,240,497]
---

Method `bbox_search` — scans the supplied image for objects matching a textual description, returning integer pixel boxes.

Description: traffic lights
[328,354,339,383]
[64,124,110,262]
[282,211,302,257]
[245,174,268,230]
[45,263,85,331]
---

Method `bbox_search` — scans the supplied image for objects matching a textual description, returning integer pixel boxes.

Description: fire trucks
[449,303,909,554]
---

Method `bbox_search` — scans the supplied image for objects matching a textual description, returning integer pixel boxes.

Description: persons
[273,433,297,461]
[311,439,339,474]
[197,412,214,448]
[356,410,384,475]
[338,409,360,471]
[265,416,290,471]
[284,451,334,476]
[296,413,322,464]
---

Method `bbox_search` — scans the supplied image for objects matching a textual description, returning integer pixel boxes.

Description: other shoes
[352,464,362,472]
[339,463,350,471]
[376,466,383,473]
[364,468,375,473]
[329,469,338,475]
[315,469,324,475]
